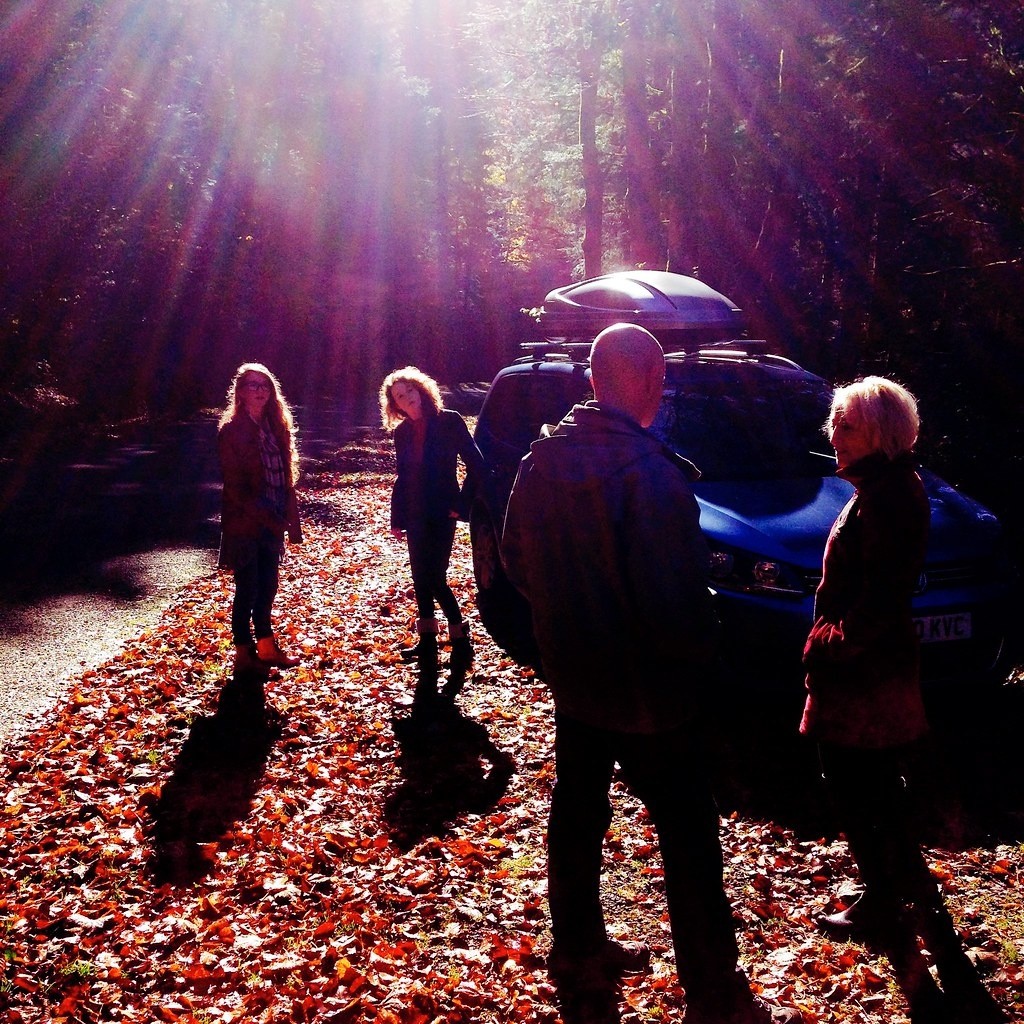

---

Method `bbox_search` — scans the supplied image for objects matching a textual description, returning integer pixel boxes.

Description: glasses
[240,380,273,394]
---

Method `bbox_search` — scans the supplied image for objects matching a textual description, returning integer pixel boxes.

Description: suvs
[455,268,1000,646]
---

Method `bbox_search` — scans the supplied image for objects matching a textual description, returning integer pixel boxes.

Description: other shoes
[681,1000,804,1024]
[818,886,905,939]
[899,857,944,912]
[548,934,646,981]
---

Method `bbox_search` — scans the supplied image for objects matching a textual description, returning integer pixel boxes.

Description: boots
[448,617,476,665]
[233,640,279,680]
[256,633,301,667]
[401,618,440,657]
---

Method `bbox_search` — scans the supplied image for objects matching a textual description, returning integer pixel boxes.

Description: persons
[500,322,942,1024]
[216,362,302,679]
[379,366,485,662]
